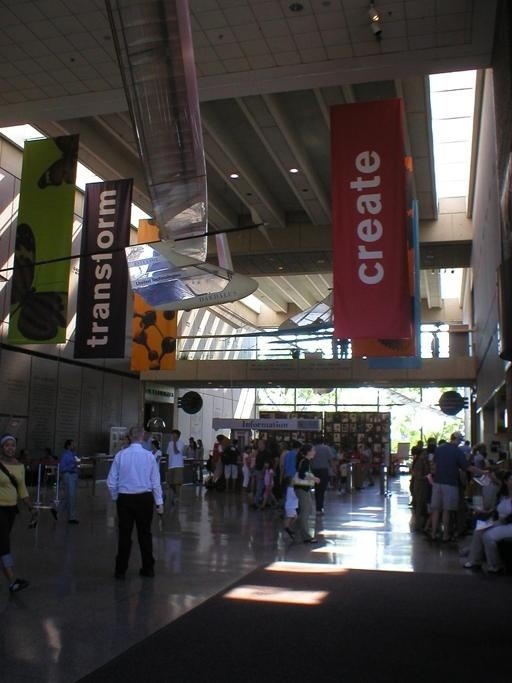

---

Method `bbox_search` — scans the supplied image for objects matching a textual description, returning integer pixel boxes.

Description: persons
[1,432,41,596]
[104,424,166,581]
[119,434,133,451]
[409,431,511,574]
[430,331,440,357]
[280,474,301,528]
[142,429,157,451]
[188,433,352,512]
[165,427,186,505]
[284,443,322,544]
[49,436,83,526]
[339,338,350,359]
[353,438,375,489]
[149,439,163,465]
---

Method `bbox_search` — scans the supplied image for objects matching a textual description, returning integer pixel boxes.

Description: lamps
[368,0,382,43]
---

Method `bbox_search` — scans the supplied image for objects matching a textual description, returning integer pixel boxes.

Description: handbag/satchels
[17,495,38,525]
[293,458,315,487]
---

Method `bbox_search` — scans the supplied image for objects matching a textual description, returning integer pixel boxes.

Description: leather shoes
[139,569,154,579]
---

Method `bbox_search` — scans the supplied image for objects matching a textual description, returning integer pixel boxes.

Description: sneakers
[50,508,57,520]
[68,520,79,524]
[8,578,30,593]
[458,541,505,573]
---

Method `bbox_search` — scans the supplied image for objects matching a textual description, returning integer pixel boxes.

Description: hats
[451,432,465,441]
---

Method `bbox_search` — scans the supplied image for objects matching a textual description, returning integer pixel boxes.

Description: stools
[502,537,512,574]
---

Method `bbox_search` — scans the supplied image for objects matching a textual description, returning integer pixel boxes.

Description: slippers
[285,527,296,540]
[303,538,318,543]
[422,528,458,542]
